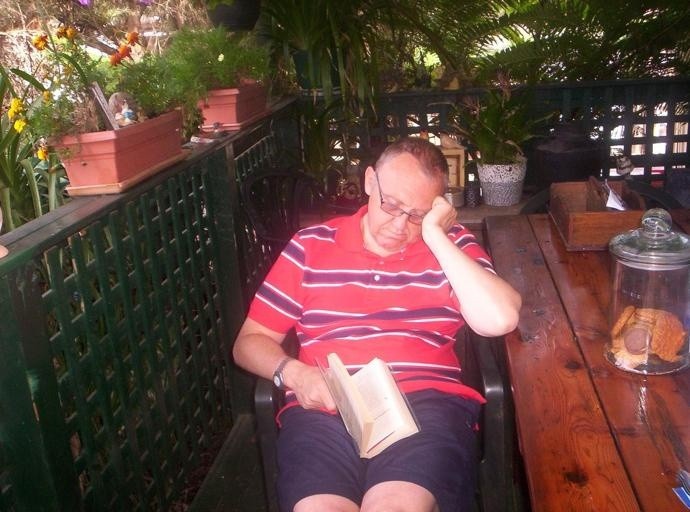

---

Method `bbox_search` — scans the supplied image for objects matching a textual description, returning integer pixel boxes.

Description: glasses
[375,171,424,225]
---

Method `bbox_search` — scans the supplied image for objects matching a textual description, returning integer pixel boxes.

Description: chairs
[238,167,359,257]
[251,334,507,512]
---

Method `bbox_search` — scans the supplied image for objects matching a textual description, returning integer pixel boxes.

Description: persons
[232,136,523,512]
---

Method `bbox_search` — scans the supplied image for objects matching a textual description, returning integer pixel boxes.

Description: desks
[481,207,690,512]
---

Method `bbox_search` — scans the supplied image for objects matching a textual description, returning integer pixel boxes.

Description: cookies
[612,305,686,370]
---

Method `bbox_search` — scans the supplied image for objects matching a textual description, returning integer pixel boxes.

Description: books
[314,350,422,461]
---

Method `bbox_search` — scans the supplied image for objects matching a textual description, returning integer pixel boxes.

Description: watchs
[272,356,295,392]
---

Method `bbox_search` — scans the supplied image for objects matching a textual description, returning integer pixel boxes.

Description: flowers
[7,20,276,163]
[425,66,560,164]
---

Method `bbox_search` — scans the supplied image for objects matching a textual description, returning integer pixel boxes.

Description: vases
[477,166,526,209]
[42,104,185,189]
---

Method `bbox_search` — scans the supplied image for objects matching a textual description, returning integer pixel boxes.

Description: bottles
[602,207,690,377]
